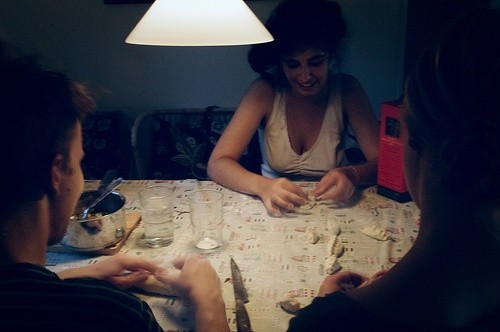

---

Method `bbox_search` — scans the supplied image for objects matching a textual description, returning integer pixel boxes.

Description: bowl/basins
[63,189,126,249]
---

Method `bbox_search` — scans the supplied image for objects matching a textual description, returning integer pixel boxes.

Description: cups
[139,185,174,247]
[190,190,223,249]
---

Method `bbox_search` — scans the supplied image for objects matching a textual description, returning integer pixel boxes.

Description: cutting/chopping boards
[44,211,142,255]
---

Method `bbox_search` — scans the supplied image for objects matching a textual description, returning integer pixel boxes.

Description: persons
[0,60,230,332]
[206,0,380,217]
[288,50,500,332]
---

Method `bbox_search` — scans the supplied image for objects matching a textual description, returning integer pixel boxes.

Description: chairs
[130,107,263,180]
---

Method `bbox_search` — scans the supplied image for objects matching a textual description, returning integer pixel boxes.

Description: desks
[45,179,422,332]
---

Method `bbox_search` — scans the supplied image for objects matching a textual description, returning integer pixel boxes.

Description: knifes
[231,258,251,332]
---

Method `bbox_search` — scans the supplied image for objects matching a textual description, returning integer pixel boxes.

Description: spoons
[69,178,123,219]
